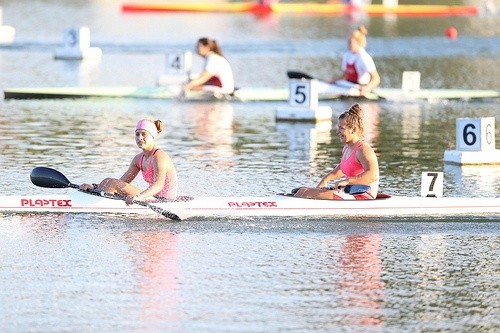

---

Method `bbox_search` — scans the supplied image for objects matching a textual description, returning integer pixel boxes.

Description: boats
[0,172,500,221]
[3,72,500,103]
[121,0,478,18]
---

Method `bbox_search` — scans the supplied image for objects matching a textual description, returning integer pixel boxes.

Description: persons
[294,104,379,200]
[79,119,177,206]
[179,38,234,97]
[330,26,381,97]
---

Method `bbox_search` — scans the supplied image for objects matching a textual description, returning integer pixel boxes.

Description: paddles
[287,71,362,91]
[30,167,183,222]
[292,183,371,194]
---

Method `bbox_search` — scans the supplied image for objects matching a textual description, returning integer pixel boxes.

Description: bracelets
[92,184,97,189]
